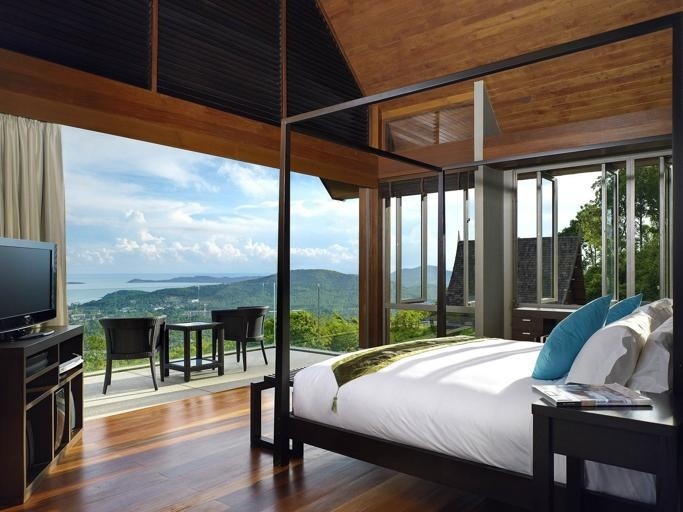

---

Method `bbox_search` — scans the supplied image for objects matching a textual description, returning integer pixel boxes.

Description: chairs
[98,315,167,395]
[212,306,270,372]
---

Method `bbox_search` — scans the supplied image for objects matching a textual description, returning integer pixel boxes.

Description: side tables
[166,320,224,382]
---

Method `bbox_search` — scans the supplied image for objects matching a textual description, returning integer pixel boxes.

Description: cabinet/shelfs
[0,323,86,507]
[512,309,543,342]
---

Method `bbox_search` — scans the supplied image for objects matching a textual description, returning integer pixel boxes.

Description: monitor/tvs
[0,238,58,338]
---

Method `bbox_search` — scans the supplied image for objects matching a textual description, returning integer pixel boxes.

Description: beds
[276,11,683,512]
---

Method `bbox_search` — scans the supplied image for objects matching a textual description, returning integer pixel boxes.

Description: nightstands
[532,392,683,512]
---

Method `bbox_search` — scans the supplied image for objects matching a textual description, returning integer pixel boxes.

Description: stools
[251,367,314,458]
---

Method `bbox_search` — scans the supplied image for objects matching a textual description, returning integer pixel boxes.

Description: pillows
[566,306,656,386]
[631,316,674,393]
[605,292,644,324]
[533,291,612,380]
[635,298,673,332]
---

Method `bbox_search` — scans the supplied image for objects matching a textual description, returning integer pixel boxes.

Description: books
[59,353,84,374]
[531,382,651,408]
